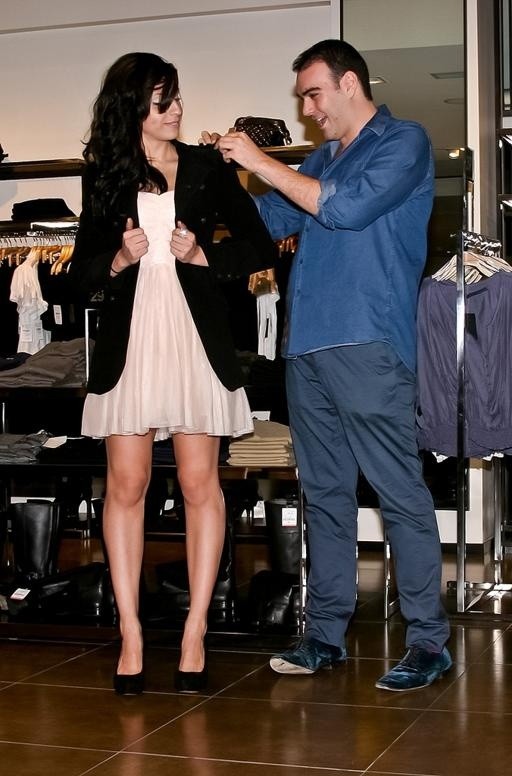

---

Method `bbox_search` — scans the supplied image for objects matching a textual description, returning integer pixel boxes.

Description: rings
[179,228,188,238]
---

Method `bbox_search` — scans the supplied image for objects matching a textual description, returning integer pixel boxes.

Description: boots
[8,498,297,628]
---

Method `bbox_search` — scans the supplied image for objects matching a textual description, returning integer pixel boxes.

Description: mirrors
[338,0,470,512]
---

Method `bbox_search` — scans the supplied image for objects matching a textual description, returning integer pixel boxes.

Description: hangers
[429,229,511,287]
[0,231,79,277]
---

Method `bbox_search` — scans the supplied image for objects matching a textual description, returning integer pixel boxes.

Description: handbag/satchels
[236,117,294,147]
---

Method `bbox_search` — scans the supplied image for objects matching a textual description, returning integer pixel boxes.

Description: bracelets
[110,268,121,275]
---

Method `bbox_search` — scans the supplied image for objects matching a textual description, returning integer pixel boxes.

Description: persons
[71,50,280,697]
[198,38,452,693]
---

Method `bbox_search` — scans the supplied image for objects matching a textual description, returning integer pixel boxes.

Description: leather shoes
[269,637,340,677]
[376,646,451,692]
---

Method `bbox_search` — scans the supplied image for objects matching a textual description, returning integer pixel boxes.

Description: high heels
[112,660,145,694]
[173,648,205,693]
